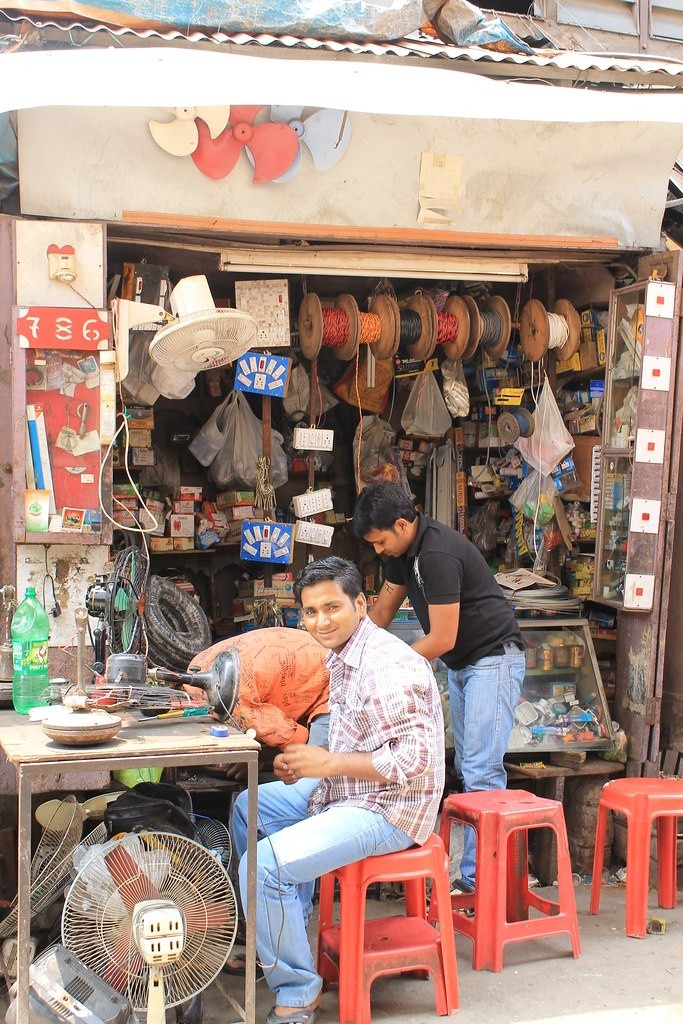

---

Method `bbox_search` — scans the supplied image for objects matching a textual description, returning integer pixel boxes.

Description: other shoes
[448,880,476,917]
[237,930,247,945]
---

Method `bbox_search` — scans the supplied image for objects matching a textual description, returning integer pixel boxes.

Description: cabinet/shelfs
[385,619,617,759]
[462,281,607,600]
[593,280,676,613]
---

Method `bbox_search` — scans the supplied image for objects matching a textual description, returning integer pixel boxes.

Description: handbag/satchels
[104,782,195,839]
[188,387,289,492]
[512,372,575,478]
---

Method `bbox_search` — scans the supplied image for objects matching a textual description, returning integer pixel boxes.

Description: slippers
[265,1007,319,1024]
[222,953,265,978]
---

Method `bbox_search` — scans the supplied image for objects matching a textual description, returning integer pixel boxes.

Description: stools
[317,832,461,1024]
[589,777,683,939]
[429,788,582,972]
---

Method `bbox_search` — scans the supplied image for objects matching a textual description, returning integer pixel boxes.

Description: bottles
[11,587,50,714]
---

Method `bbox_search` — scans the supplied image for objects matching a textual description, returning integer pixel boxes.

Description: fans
[38,649,241,723]
[0,783,239,1024]
[85,546,146,684]
[111,273,260,384]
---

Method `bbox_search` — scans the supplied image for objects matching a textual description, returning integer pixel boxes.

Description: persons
[221,556,445,1024]
[170,626,331,943]
[353,479,526,918]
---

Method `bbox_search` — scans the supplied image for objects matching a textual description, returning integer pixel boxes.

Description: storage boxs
[463,309,607,553]
[113,402,300,631]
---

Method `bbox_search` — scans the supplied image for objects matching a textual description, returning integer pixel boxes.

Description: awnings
[0,9,682,121]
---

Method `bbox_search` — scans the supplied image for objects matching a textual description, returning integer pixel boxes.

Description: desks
[0,709,262,1024]
